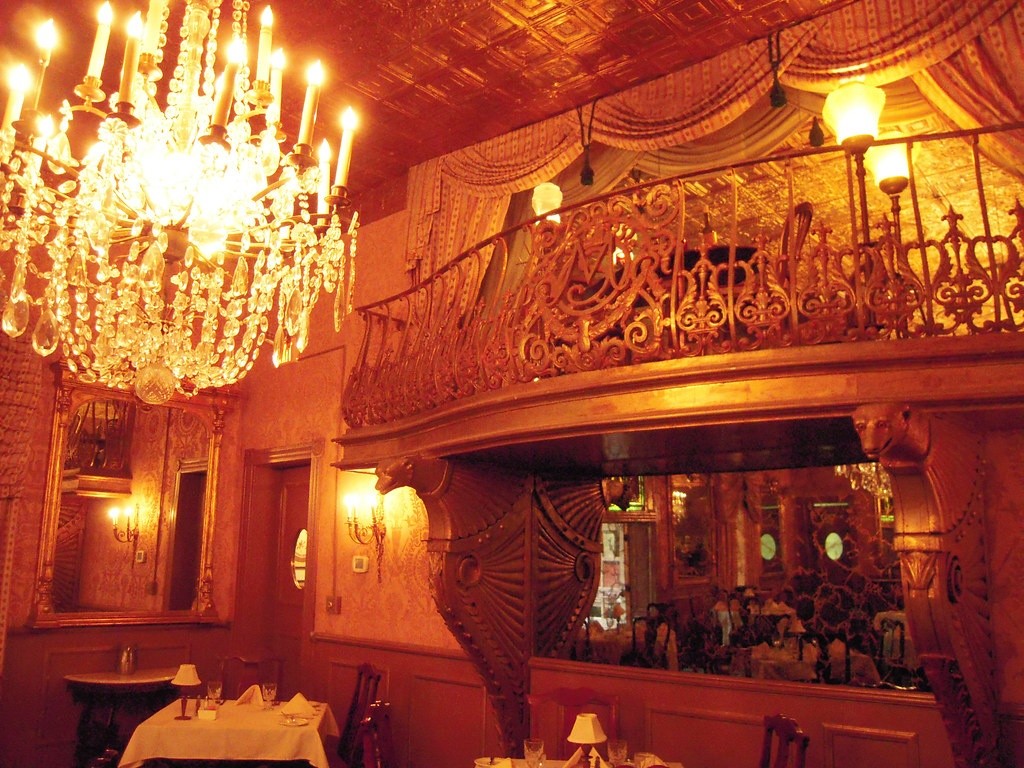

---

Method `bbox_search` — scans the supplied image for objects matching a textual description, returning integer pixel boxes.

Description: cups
[635,752,655,768]
[608,740,627,768]
[524,738,544,768]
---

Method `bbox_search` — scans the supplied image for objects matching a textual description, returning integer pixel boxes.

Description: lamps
[170,663,202,720]
[107,504,137,543]
[820,79,886,273]
[0,0,360,406]
[342,488,387,544]
[835,463,892,512]
[567,711,608,767]
[866,132,917,339]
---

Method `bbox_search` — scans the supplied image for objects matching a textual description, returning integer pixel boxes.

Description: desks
[65,668,183,768]
[585,244,770,347]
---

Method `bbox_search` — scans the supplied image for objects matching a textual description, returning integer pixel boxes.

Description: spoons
[281,712,295,723]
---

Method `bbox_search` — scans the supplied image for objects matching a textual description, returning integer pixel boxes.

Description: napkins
[234,685,265,706]
[489,757,513,768]
[279,693,317,717]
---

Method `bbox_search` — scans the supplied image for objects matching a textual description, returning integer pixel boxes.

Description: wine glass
[262,684,276,711]
[606,621,614,633]
[208,682,221,706]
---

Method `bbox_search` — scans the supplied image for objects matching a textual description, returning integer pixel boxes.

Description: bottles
[702,213,716,247]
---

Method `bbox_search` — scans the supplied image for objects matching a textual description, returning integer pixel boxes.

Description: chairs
[216,653,392,768]
[573,583,905,686]
[720,202,814,333]
[757,713,810,768]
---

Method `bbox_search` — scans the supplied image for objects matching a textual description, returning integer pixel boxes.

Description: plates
[282,718,309,725]
[474,757,505,767]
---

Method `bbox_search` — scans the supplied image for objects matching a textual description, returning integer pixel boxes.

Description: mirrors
[24,357,242,627]
[530,471,929,697]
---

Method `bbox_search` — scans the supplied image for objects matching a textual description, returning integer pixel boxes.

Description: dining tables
[709,603,803,646]
[875,611,905,658]
[731,639,879,694]
[589,621,677,671]
[117,697,340,768]
[477,758,682,768]
